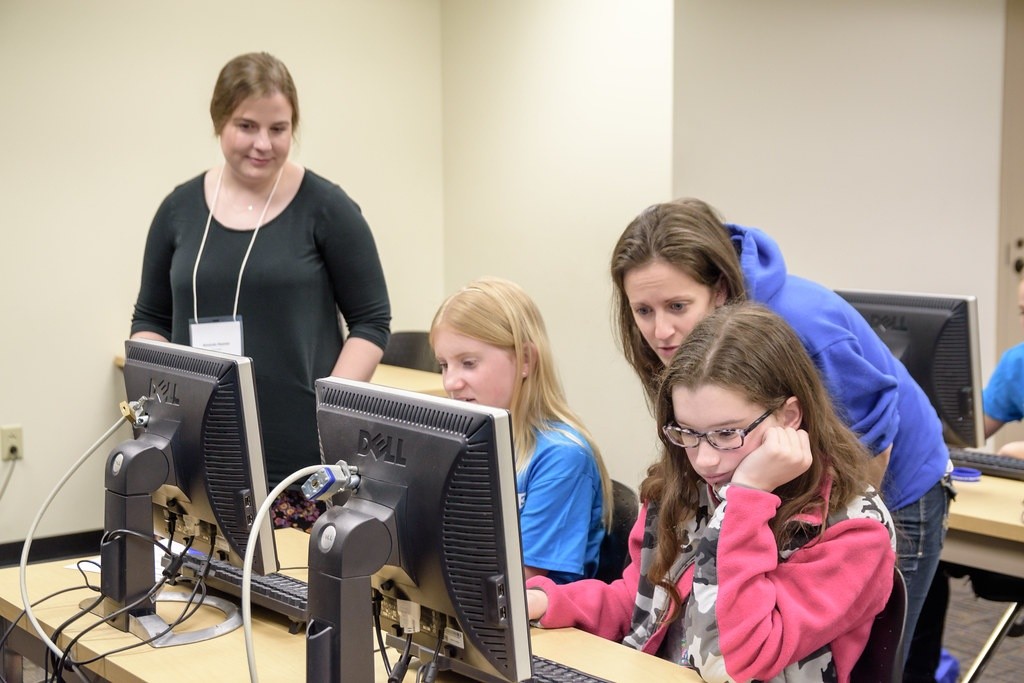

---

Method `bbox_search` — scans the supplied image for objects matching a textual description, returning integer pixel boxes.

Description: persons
[982,267,1024,460]
[525,302,897,683]
[429,279,615,583]
[129,51,391,532]
[611,197,958,683]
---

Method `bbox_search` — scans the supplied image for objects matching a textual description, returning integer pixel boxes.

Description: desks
[938,474,1024,579]
[370,364,449,397]
[0,527,706,683]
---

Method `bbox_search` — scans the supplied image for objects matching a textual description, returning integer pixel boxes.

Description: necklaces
[246,202,253,210]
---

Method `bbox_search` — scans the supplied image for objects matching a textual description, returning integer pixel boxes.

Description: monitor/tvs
[78,336,280,648]
[833,288,986,449]
[307,376,533,683]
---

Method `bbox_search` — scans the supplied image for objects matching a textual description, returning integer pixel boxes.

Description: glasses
[663,408,774,451]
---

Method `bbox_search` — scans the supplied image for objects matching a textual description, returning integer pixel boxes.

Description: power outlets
[0,427,23,461]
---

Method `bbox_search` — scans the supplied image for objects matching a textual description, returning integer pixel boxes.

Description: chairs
[850,566,908,683]
[380,332,442,374]
[596,479,639,585]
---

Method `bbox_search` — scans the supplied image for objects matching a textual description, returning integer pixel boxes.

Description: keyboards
[531,654,616,683]
[161,552,309,635]
[947,449,1024,482]
